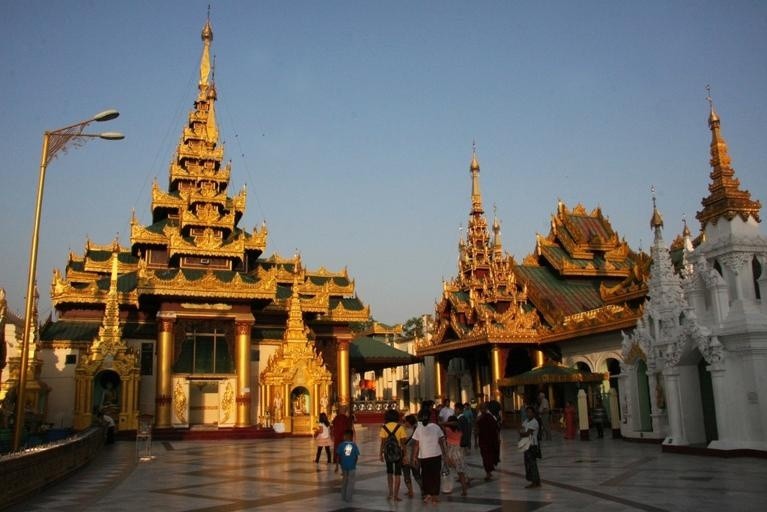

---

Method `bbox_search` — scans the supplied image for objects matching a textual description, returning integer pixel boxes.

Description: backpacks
[383,423,403,462]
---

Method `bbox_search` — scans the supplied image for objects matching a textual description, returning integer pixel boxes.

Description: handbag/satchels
[517,438,531,452]
[441,472,453,493]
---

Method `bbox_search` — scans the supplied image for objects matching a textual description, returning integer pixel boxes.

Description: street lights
[13,105,126,457]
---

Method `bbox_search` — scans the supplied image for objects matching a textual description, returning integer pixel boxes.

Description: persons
[518,406,542,489]
[520,391,594,441]
[331,406,355,474]
[96,412,115,447]
[378,395,502,505]
[312,412,332,469]
[98,379,119,421]
[336,430,361,503]
